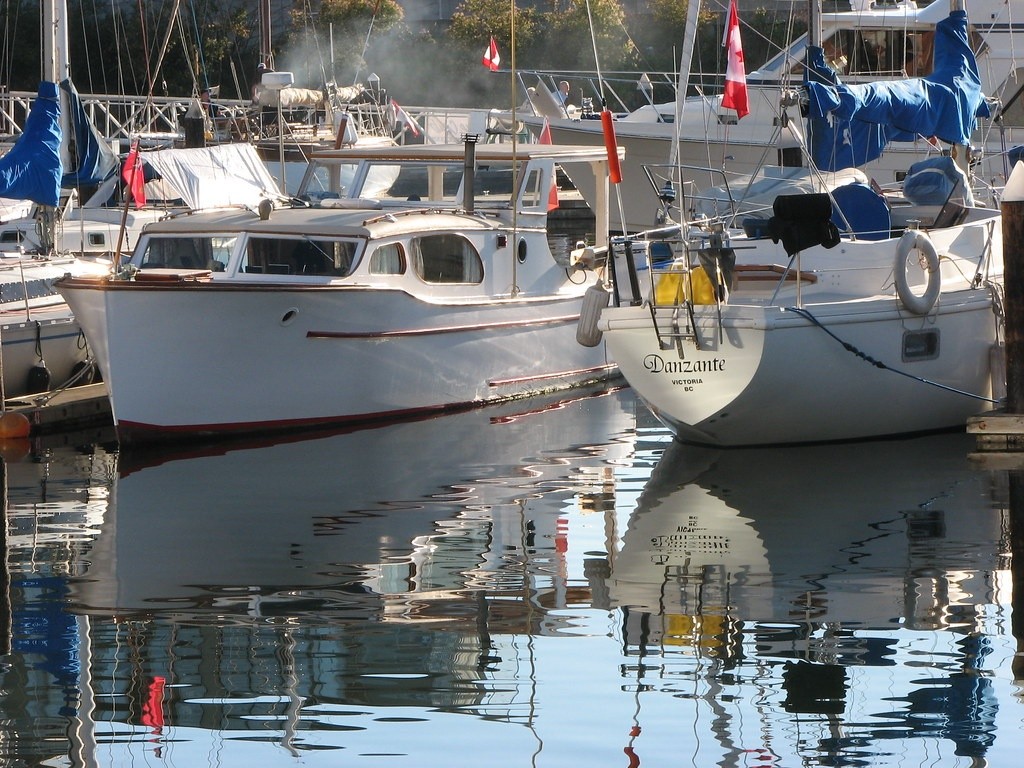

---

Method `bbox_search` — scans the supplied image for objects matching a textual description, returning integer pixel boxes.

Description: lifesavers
[893,230,941,315]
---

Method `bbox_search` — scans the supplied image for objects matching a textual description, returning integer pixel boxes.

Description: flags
[482,36,500,70]
[538,117,560,212]
[390,100,418,139]
[141,675,165,727]
[122,142,146,209]
[721,0,750,120]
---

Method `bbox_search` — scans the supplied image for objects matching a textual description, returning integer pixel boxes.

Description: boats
[0,0,1024,445]
[64,374,643,618]
[600,426,1012,634]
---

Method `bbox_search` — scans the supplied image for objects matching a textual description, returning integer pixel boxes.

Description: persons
[520,87,536,112]
[552,81,569,106]
[200,90,220,118]
